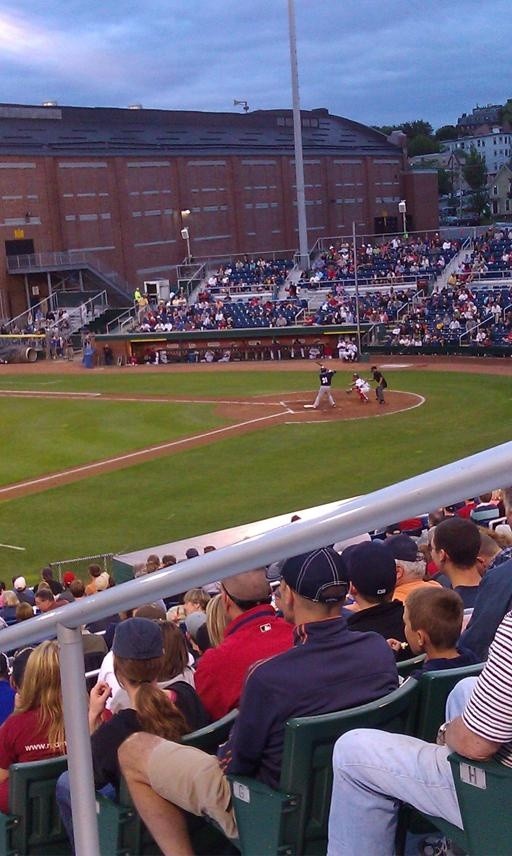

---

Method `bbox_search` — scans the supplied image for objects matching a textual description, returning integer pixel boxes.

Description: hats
[385,534,418,562]
[222,568,274,604]
[184,613,207,639]
[342,540,396,597]
[14,577,26,590]
[64,572,75,583]
[112,617,163,660]
[266,546,347,602]
[43,567,53,578]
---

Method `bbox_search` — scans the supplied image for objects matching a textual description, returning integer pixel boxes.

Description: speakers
[181,230,189,239]
[399,203,406,213]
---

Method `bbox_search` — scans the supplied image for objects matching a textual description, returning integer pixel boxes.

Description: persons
[367,366,387,405]
[346,372,371,404]
[1,296,113,368]
[127,227,511,368]
[1,483,511,853]
[311,364,337,410]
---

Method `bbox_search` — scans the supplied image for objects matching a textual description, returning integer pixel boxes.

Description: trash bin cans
[84,348,95,368]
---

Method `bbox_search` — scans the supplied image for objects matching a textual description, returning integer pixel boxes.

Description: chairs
[3,488,509,854]
[2,231,508,363]
